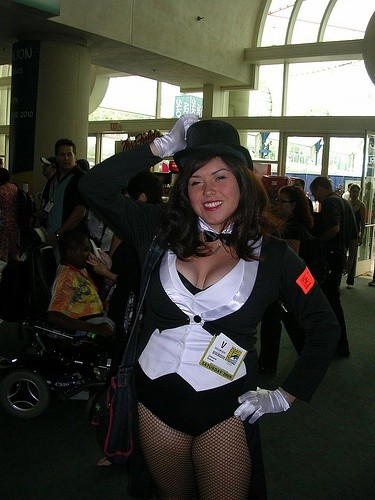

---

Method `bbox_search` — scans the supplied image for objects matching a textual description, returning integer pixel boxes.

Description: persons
[78,114,339,500]
[0,168,21,262]
[29,157,56,227]
[342,182,375,286]
[40,139,86,263]
[258,186,313,374]
[346,185,365,288]
[309,177,358,359]
[49,233,113,335]
[86,172,163,342]
[294,179,313,214]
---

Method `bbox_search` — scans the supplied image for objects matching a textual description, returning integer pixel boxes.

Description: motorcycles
[0,310,127,422]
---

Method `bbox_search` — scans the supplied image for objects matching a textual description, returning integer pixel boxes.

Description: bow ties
[203,230,234,247]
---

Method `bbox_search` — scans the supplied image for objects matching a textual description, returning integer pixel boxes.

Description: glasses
[275,197,292,204]
[42,163,51,167]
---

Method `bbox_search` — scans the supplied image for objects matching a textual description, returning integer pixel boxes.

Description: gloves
[234,385,292,424]
[152,112,201,159]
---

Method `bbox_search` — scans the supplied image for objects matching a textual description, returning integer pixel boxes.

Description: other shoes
[368,280,375,287]
[346,283,354,289]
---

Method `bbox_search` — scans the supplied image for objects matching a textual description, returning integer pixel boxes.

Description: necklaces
[198,218,234,246]
[212,244,221,254]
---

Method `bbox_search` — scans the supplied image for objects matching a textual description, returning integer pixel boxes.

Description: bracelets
[55,232,61,240]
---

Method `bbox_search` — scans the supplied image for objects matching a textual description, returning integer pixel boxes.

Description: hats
[39,156,56,165]
[174,119,254,171]
